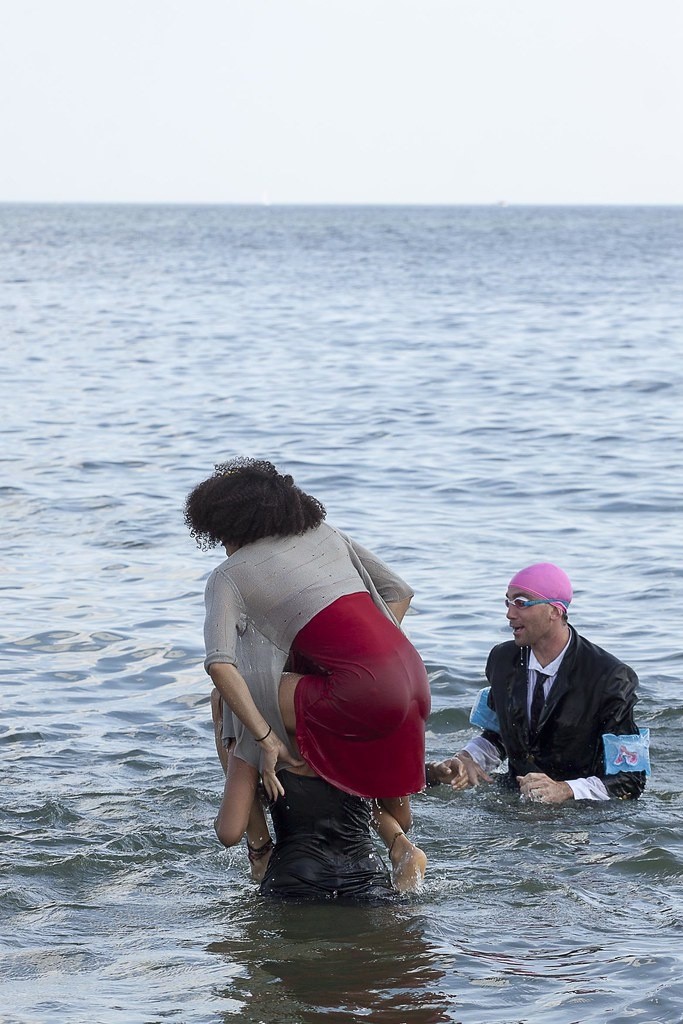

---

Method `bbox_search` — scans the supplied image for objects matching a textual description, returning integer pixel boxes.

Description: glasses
[504,596,531,609]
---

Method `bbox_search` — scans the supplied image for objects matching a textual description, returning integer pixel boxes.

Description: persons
[184,456,469,894]
[456,562,646,803]
[214,739,412,894]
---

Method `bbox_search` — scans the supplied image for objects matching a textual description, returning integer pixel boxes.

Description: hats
[509,563,572,612]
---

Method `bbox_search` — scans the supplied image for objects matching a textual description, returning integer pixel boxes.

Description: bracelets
[254,724,272,741]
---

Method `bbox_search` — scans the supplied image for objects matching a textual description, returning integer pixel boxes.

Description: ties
[530,670,550,746]
